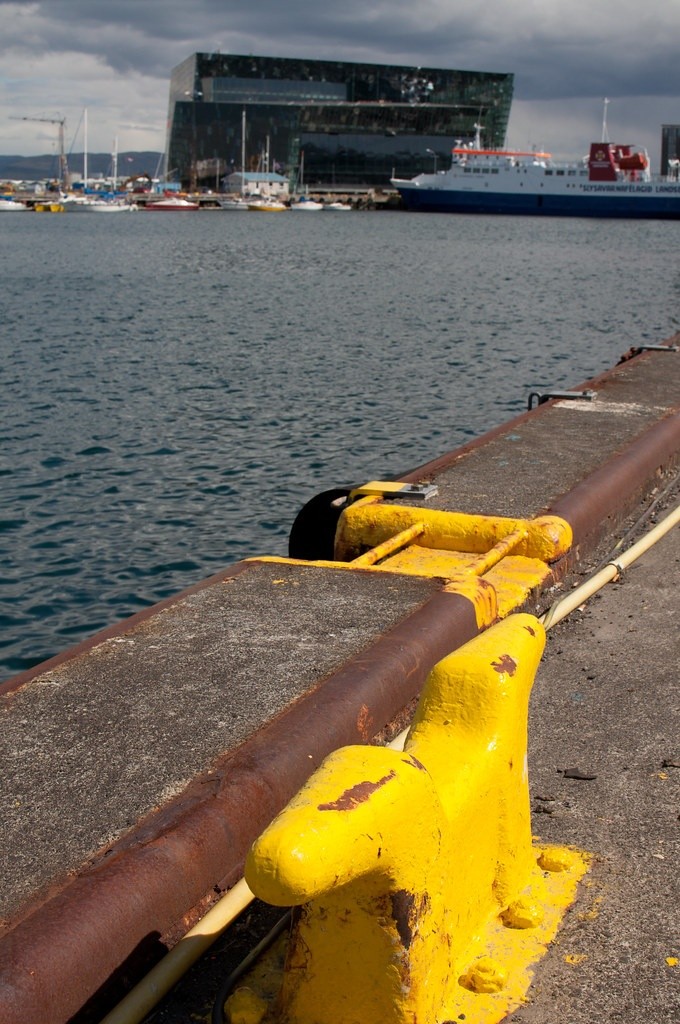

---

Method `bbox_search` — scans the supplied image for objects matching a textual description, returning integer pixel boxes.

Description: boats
[389,97,679,217]
[0,109,402,212]
